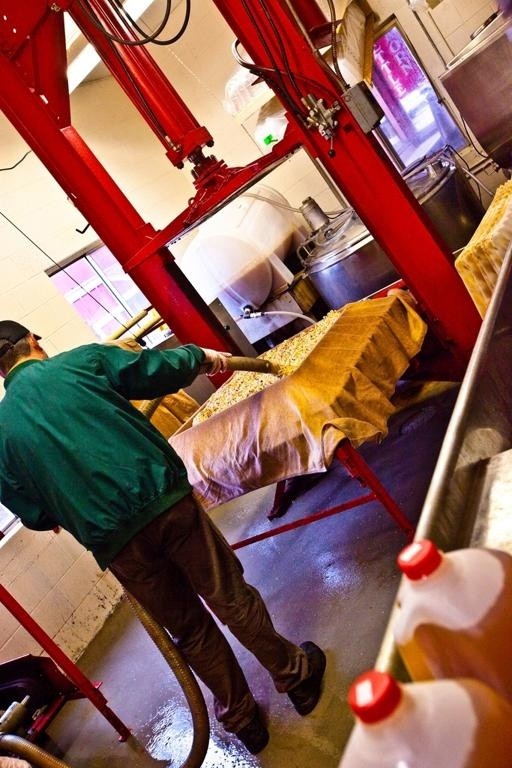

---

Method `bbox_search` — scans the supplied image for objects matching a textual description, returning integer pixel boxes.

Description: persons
[0,318,325,753]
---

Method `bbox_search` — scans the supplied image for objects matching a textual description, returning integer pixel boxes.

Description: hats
[0,321,42,355]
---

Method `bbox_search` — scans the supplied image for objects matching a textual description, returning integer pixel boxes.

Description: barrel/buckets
[335,676,512,767]
[395,541,512,695]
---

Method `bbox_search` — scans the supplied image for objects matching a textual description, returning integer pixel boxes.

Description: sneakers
[236,718,268,756]
[288,641,326,716]
